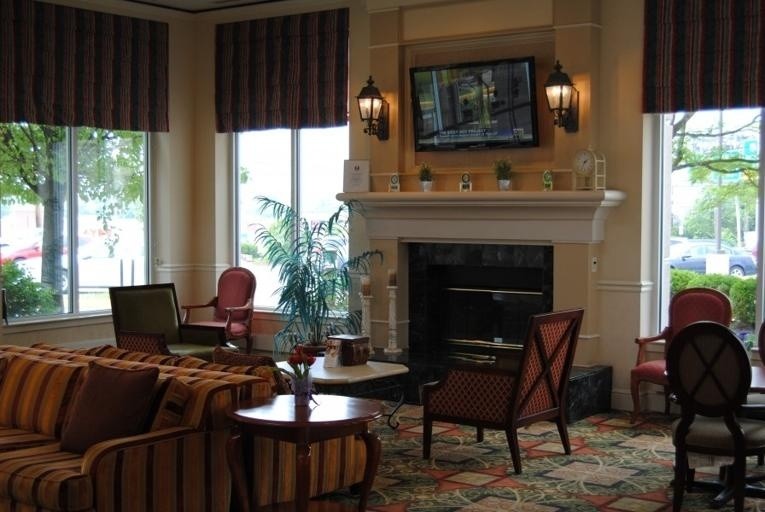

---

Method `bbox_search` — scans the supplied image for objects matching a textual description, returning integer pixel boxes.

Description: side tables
[224,393,384,512]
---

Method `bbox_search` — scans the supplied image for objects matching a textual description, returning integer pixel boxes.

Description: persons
[102,223,122,258]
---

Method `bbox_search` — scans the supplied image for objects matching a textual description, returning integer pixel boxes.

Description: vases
[293,376,312,407]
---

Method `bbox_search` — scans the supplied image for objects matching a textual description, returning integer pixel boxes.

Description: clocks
[571,146,607,192]
[388,174,400,192]
[543,170,553,191]
[458,173,472,192]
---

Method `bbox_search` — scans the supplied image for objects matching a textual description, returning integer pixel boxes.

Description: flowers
[263,343,316,381]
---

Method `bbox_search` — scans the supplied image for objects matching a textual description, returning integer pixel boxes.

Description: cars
[668,240,757,286]
[1,211,145,306]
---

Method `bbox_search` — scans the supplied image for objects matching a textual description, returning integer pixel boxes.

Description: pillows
[58,359,158,455]
[213,345,293,395]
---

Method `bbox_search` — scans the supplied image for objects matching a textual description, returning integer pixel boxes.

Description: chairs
[180,267,256,355]
[109,281,228,363]
[422,309,585,475]
[629,287,765,512]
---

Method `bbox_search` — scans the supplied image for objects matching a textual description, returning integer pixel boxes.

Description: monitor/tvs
[409,56,539,152]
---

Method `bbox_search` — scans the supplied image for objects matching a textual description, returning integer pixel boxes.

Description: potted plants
[417,159,433,192]
[248,196,384,360]
[493,158,513,192]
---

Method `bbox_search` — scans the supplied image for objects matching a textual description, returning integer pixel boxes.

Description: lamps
[355,75,389,141]
[543,59,579,133]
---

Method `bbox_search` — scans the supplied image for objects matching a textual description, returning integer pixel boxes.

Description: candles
[388,268,397,286]
[360,275,372,296]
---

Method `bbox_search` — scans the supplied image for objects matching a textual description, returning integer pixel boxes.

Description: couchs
[32,342,367,506]
[0,351,237,512]
